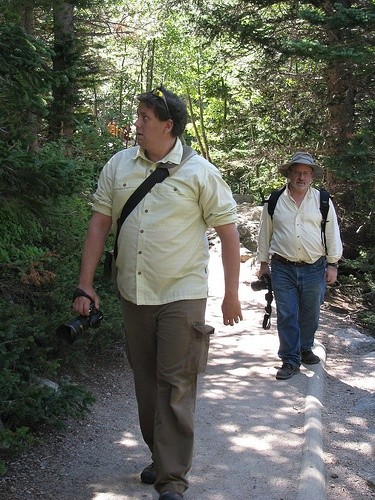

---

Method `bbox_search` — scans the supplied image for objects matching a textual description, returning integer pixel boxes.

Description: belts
[272,254,305,266]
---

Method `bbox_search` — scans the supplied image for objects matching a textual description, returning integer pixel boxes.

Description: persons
[71,85,243,500]
[257,152,343,379]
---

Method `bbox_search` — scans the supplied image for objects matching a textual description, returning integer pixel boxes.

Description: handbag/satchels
[103,249,118,287]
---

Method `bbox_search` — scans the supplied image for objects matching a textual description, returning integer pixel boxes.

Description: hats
[278,152,323,180]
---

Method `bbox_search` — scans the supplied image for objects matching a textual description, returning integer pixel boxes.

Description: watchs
[327,263,339,269]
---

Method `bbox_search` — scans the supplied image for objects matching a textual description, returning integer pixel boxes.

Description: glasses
[152,89,171,119]
[291,169,311,178]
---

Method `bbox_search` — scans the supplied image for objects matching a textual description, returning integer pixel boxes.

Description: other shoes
[302,351,320,365]
[158,490,183,500]
[140,462,156,484]
[276,360,301,379]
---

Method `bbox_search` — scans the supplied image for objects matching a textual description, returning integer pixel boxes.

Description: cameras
[251,274,273,291]
[55,307,103,344]
